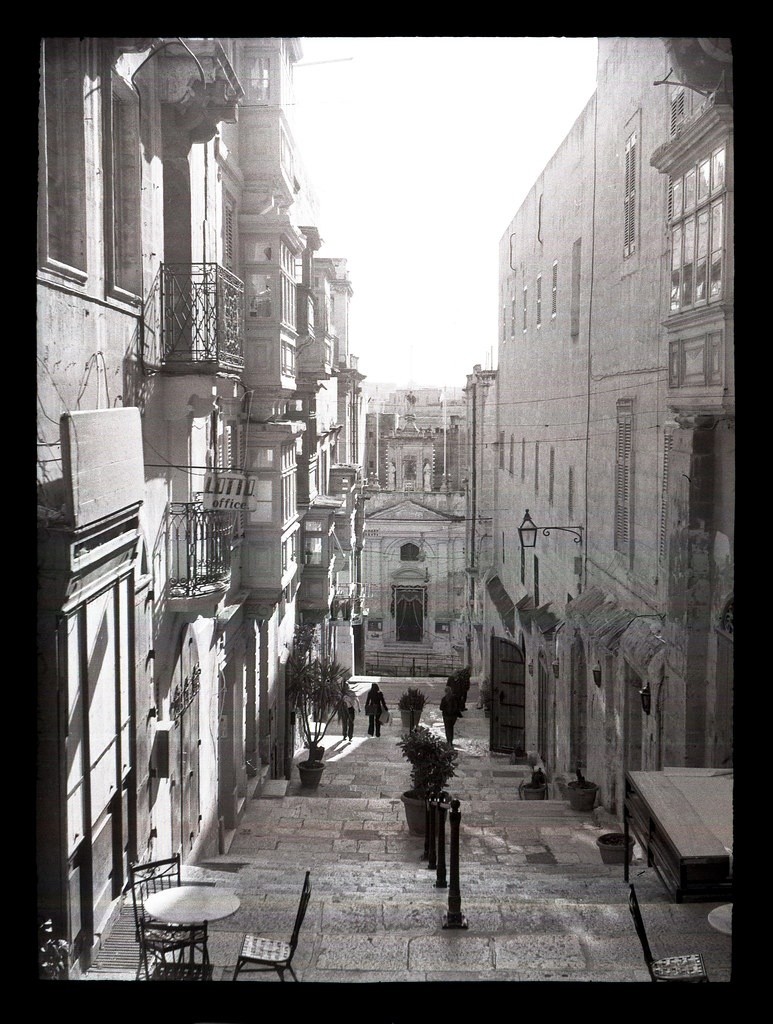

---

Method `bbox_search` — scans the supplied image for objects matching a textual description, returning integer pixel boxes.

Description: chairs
[129,853,209,981]
[629,883,709,982]
[140,916,211,981]
[232,871,312,982]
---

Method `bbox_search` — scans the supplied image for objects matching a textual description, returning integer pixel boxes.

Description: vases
[596,833,636,864]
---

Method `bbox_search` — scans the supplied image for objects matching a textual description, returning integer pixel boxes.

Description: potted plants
[398,687,430,727]
[396,727,459,837]
[523,768,546,800]
[285,655,361,789]
[567,769,599,812]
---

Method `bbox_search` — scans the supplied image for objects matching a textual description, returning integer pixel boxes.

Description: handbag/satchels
[365,704,377,715]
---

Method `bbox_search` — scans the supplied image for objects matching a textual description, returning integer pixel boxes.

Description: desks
[708,903,733,936]
[144,886,240,981]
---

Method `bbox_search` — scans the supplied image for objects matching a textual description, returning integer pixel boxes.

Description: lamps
[517,509,583,547]
[592,660,601,688]
[552,657,559,678]
[639,682,651,715]
[528,659,534,677]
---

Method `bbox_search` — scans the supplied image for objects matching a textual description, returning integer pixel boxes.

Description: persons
[365,683,388,738]
[458,664,471,712]
[440,686,459,747]
[338,682,361,742]
[446,668,465,718]
